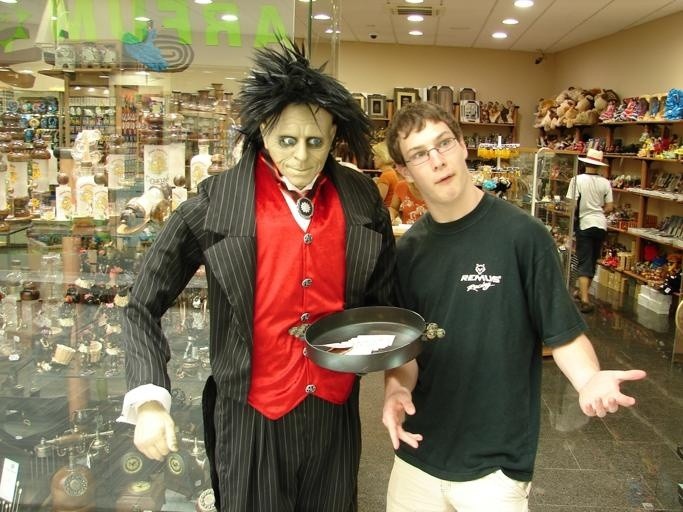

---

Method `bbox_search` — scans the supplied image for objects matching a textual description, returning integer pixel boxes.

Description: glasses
[396,133,461,168]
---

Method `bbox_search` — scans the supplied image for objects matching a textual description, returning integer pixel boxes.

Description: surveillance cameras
[535,49,545,65]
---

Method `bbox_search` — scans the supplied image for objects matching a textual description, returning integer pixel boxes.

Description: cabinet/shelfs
[532,117,683,363]
[0,67,243,188]
[335,99,578,361]
[364,106,519,175]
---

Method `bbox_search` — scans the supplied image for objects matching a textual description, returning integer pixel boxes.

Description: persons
[378,100,650,512]
[386,164,427,227]
[369,140,400,209]
[112,25,410,512]
[564,145,617,313]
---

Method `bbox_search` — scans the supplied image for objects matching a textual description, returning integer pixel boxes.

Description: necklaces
[297,191,315,220]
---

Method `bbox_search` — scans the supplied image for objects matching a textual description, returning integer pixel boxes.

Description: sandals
[573,288,595,313]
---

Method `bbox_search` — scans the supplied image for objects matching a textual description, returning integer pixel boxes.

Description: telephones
[34,388,218,512]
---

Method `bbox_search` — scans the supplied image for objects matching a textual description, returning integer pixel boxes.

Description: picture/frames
[348,85,481,124]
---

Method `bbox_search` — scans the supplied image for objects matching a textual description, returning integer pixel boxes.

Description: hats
[577,148,609,168]
